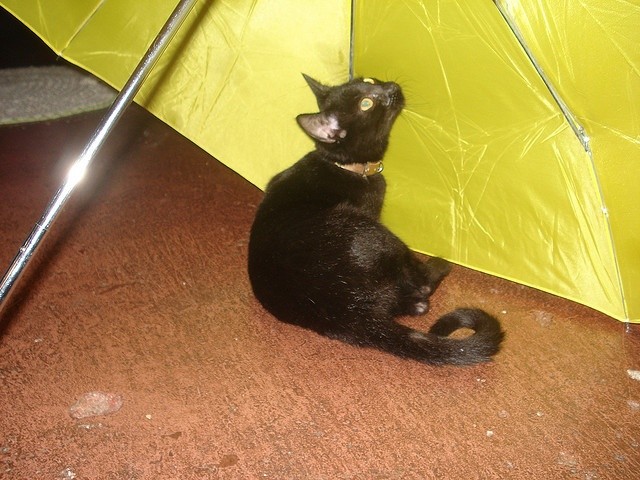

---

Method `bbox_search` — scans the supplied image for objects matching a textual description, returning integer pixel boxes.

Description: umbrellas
[0,0,640,329]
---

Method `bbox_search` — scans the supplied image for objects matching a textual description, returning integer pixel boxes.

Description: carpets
[0,65,118,126]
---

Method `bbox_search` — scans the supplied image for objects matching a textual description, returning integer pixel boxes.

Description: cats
[247,71,507,368]
